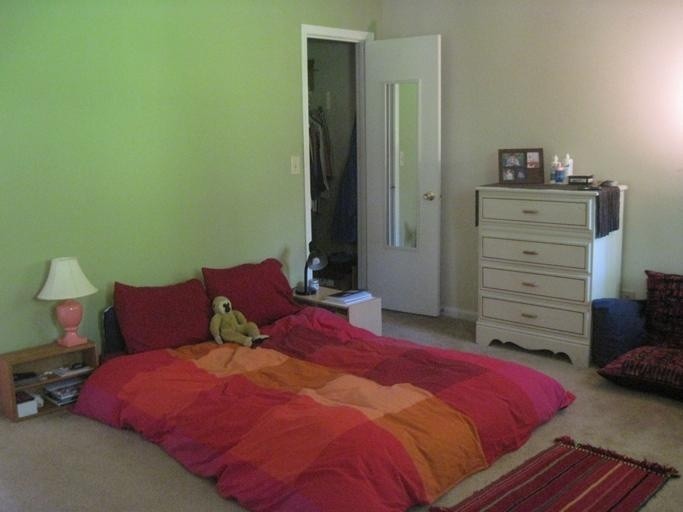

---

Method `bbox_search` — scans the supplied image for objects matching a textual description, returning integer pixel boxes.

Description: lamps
[35,257,100,348]
[296,242,329,295]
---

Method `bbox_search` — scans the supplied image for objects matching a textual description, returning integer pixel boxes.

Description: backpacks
[592,297,648,370]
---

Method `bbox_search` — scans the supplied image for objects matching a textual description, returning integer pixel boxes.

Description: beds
[69,308,575,512]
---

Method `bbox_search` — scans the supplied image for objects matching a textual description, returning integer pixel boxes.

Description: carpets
[437,435,674,512]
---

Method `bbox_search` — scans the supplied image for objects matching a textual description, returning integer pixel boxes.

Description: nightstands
[291,286,383,336]
[0,343,97,422]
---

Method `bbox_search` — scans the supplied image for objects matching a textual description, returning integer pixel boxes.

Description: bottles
[549,153,574,185]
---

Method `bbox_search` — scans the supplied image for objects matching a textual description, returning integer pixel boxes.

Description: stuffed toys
[208,295,270,347]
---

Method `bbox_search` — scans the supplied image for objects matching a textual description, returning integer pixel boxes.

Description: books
[42,376,85,407]
[324,288,374,304]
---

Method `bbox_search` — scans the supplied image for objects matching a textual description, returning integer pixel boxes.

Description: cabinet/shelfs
[475,184,629,369]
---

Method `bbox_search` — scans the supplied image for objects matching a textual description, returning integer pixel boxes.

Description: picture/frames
[498,148,545,186]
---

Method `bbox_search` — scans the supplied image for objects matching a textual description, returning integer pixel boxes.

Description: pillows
[116,280,213,353]
[593,270,683,400]
[201,258,306,326]
